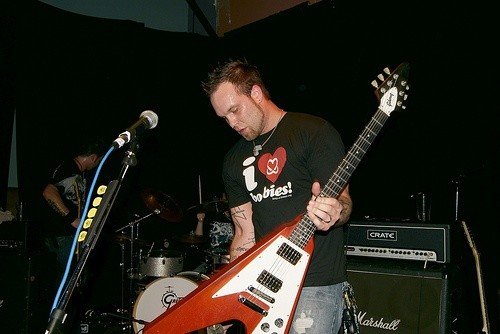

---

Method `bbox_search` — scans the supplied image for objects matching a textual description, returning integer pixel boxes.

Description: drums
[130,270,210,334]
[204,252,230,275]
[139,248,184,278]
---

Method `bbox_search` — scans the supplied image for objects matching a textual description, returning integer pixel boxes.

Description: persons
[23,138,108,304]
[201,57,352,334]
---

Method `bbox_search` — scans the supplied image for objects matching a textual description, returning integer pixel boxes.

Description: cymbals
[170,233,212,244]
[116,234,156,246]
[186,199,231,214]
[143,188,184,224]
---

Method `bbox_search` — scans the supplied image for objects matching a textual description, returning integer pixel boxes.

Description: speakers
[0,255,30,334]
[338,263,448,334]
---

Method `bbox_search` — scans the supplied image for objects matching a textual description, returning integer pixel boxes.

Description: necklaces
[252,109,284,156]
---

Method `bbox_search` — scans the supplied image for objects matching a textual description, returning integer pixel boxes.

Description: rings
[325,217,331,223]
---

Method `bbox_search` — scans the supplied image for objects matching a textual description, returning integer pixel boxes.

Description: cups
[16,201,25,220]
[0,199,7,211]
[449,181,463,222]
[415,193,432,221]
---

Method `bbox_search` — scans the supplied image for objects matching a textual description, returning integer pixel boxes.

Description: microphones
[110,110,158,150]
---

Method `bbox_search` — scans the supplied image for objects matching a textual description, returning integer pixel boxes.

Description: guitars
[133,62,411,334]
[461,220,491,334]
